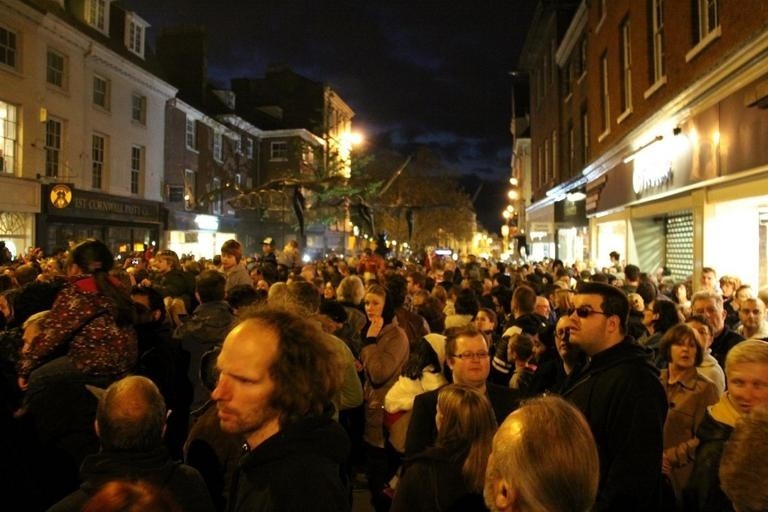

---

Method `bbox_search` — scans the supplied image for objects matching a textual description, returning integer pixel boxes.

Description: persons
[1,236,768,510]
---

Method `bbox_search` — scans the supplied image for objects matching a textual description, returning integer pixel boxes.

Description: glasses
[567,307,604,319]
[450,350,488,360]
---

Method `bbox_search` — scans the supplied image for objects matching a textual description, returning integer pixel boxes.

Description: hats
[259,237,276,247]
